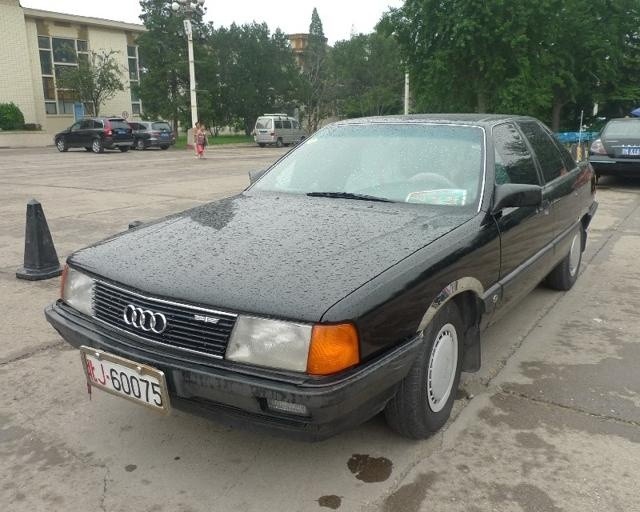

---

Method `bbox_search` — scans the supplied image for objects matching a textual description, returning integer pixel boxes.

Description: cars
[43,112,598,443]
[587,117,640,182]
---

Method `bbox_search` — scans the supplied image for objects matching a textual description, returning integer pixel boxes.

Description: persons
[196,124,209,160]
[191,122,201,161]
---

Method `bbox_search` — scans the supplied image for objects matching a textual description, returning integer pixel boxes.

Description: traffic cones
[16,199,64,280]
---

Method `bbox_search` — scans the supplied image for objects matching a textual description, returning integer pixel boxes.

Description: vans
[129,121,176,150]
[253,114,307,147]
[55,117,136,153]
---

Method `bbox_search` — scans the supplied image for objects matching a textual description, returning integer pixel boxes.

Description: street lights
[171,0,207,128]
